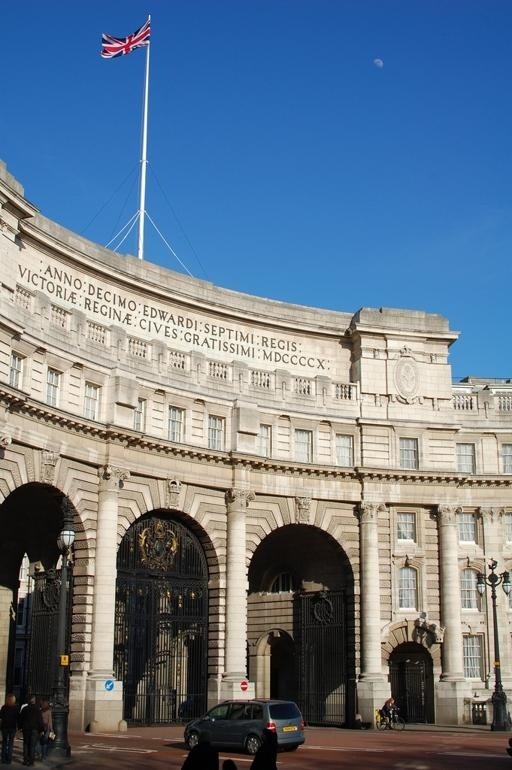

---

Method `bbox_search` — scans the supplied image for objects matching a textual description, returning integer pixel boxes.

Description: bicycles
[376,710,404,731]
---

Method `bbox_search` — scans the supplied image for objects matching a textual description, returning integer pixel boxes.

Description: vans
[184,698,304,755]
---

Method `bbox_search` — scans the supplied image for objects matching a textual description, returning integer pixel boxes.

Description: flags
[99,20,151,59]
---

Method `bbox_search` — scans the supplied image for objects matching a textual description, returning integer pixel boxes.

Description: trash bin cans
[472,701,486,725]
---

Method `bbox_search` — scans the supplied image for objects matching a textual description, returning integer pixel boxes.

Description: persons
[250,728,279,770]
[17,695,42,766]
[180,730,220,770]
[20,696,29,713]
[1,694,20,764]
[37,700,52,760]
[223,758,238,770]
[382,698,400,730]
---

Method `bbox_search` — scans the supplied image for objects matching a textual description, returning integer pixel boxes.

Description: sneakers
[1,754,49,768]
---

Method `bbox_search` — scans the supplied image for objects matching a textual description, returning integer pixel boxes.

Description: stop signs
[240,681,248,690]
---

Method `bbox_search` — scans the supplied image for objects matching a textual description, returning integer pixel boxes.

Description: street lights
[476,558,512,731]
[46,513,76,757]
